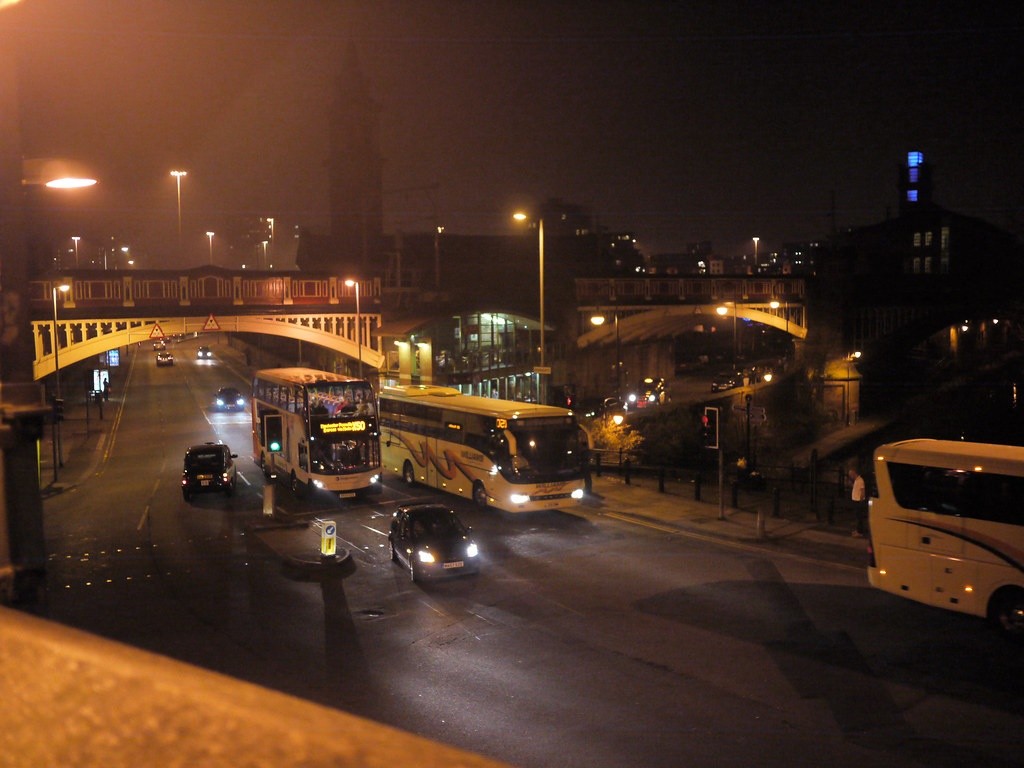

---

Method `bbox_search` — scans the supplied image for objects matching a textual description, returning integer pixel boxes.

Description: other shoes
[851,530,865,538]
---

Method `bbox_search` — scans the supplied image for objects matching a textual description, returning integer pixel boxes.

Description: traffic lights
[52,395,64,425]
[702,407,719,449]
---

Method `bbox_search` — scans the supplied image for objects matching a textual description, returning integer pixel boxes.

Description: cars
[156,353,173,366]
[388,502,479,583]
[197,347,211,359]
[584,378,669,420]
[713,357,776,394]
[214,387,244,412]
[154,334,183,350]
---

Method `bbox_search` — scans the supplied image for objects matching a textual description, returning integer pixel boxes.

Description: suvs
[182,443,238,501]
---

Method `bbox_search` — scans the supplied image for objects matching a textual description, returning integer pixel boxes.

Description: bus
[866,438,1024,637]
[250,367,382,496]
[375,384,594,515]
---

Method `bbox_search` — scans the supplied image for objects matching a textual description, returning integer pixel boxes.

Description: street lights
[171,169,186,268]
[345,280,364,378]
[53,285,71,468]
[770,279,789,354]
[514,213,544,404]
[717,285,738,386]
[590,312,621,414]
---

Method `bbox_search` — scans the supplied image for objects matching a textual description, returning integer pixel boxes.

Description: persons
[848,469,866,539]
[304,397,357,420]
[103,378,111,409]
